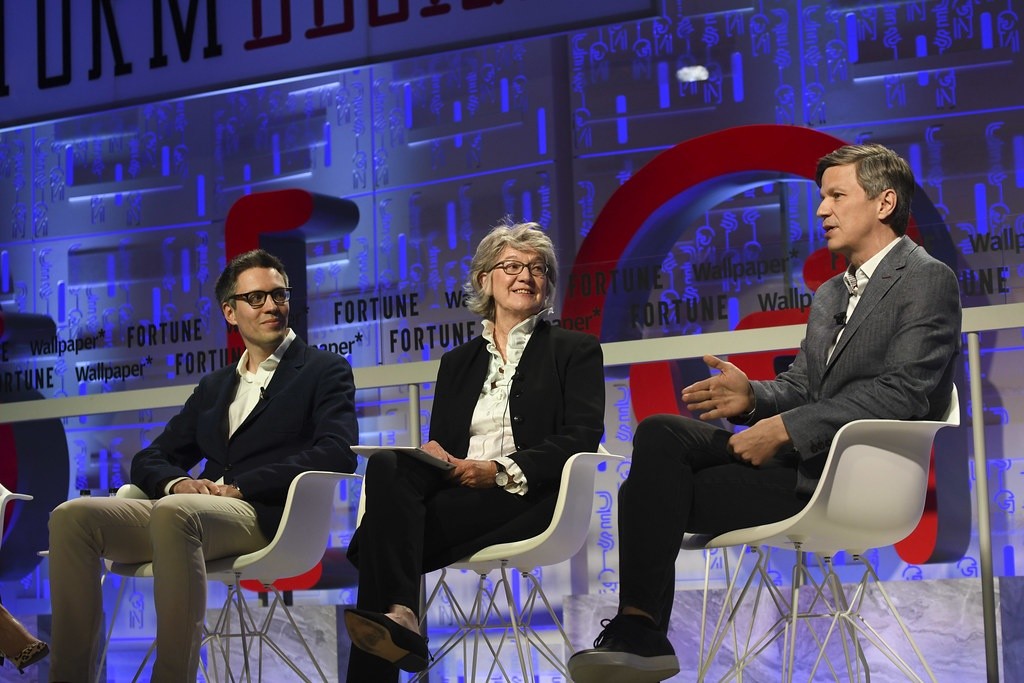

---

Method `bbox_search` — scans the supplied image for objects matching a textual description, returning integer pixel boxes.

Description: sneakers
[567,612,681,681]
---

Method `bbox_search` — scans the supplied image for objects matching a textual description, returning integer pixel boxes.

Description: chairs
[681,379,962,682]
[93,470,361,682]
[353,450,629,683]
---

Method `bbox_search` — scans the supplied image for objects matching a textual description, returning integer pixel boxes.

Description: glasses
[227,286,292,307]
[486,259,550,277]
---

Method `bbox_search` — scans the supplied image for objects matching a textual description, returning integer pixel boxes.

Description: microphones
[510,366,521,384]
[260,386,269,400]
[834,312,845,325]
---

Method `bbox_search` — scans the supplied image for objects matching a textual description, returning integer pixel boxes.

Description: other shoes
[343,608,429,673]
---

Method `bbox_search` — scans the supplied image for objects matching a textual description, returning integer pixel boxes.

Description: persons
[344,215,606,682]
[0,603,49,674]
[569,144,961,682]
[48,250,360,683]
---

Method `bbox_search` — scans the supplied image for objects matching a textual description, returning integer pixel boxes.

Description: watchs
[232,480,241,491]
[493,460,510,489]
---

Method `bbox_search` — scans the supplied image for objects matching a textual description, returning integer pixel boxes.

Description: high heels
[0,640,49,671]
